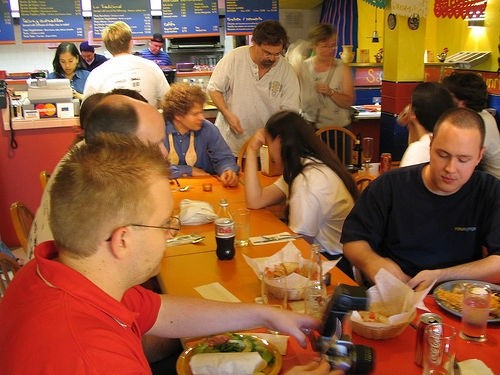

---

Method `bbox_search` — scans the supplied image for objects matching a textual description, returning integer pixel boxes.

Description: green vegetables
[191,333,272,363]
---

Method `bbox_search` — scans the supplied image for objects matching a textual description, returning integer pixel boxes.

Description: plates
[175,337,283,375]
[434,280,500,322]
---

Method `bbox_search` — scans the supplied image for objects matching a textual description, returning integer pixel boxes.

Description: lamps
[468,19,484,27]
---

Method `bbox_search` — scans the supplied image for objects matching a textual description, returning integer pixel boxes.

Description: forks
[318,317,342,364]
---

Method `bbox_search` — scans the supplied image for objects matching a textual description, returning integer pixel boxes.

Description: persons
[158,82,239,188]
[82,20,172,112]
[47,39,107,101]
[397,71,500,182]
[24,89,167,263]
[242,111,360,257]
[341,105,500,298]
[142,33,176,83]
[291,24,354,166]
[205,22,301,172]
[0,132,323,375]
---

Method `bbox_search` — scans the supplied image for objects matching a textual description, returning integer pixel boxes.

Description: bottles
[214,199,235,260]
[305,243,328,324]
[352,133,362,172]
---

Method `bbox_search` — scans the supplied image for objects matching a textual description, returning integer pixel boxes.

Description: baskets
[350,313,417,340]
[262,262,327,301]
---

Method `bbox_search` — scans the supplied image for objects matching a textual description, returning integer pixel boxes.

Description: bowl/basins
[264,261,312,300]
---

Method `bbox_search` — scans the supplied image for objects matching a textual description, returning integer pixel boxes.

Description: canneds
[380,153,391,174]
[414,313,444,367]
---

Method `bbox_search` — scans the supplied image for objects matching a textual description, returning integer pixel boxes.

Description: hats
[151,33,164,42]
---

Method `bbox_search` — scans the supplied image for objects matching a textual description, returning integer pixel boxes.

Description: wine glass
[362,138,374,174]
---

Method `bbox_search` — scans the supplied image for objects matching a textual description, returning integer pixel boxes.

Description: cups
[423,323,457,375]
[460,284,492,342]
[340,46,354,63]
[233,208,250,248]
[261,265,288,311]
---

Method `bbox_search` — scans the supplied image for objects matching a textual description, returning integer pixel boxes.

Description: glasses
[105,216,181,242]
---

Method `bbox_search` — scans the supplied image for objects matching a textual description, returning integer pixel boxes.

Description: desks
[279,295,500,375]
[155,175,360,353]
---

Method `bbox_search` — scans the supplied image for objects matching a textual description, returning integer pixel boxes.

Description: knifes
[253,235,304,243]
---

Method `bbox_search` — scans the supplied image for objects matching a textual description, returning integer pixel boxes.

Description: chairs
[314,126,359,170]
[0,171,52,292]
